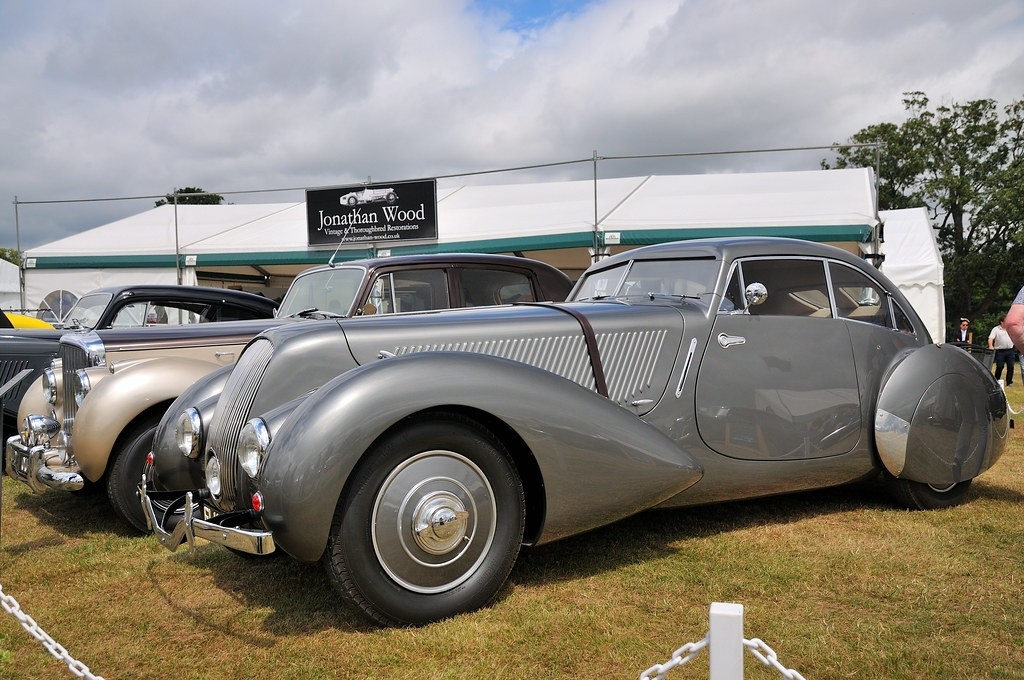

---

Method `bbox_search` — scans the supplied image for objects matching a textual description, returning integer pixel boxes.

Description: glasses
[961,324,968,326]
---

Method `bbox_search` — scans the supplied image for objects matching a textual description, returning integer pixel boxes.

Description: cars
[0,285,278,419]
[5,251,576,538]
[133,233,1010,627]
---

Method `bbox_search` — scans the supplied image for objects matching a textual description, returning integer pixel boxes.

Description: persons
[954,317,973,354]
[987,312,1017,386]
[1004,286,1024,386]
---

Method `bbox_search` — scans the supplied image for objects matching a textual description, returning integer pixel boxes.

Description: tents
[0,167,946,348]
